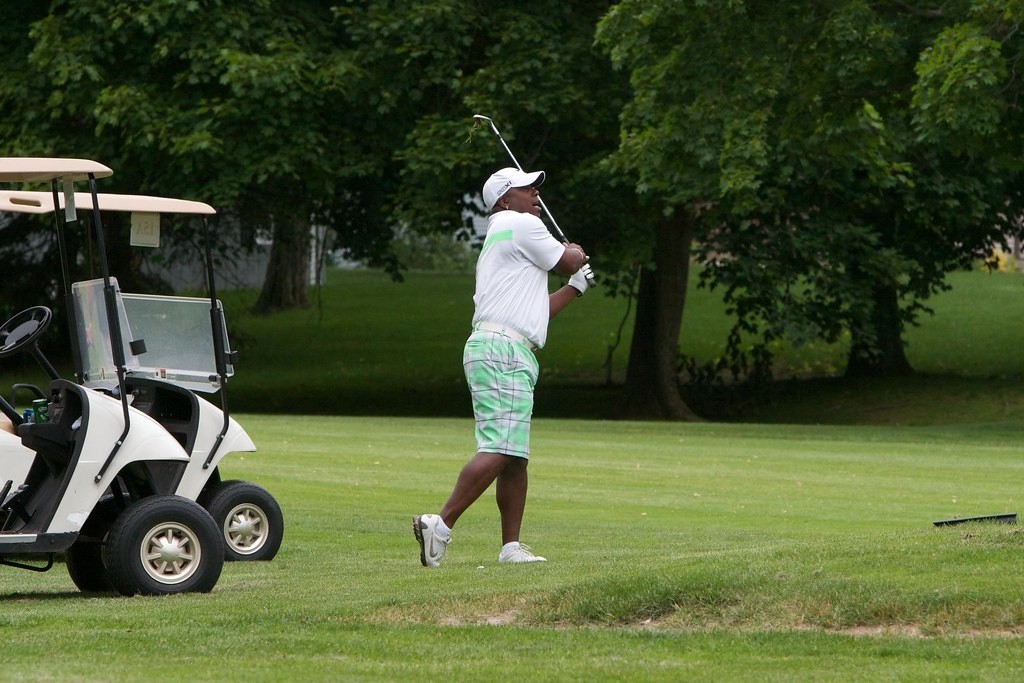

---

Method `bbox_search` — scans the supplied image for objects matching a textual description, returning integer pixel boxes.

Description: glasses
[514,185,535,193]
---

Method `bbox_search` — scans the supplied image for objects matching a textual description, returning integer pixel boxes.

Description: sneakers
[498,542,548,564]
[413,514,452,567]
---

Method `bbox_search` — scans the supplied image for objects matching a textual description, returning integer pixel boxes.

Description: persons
[413,168,596,566]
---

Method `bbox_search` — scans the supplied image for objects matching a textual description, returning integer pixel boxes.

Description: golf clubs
[472,114,598,290]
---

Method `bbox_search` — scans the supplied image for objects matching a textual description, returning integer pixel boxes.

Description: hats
[483,166,546,210]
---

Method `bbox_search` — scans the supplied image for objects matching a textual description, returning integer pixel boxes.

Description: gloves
[567,262,595,298]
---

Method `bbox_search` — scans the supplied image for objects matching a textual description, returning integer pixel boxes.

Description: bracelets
[574,248,584,260]
